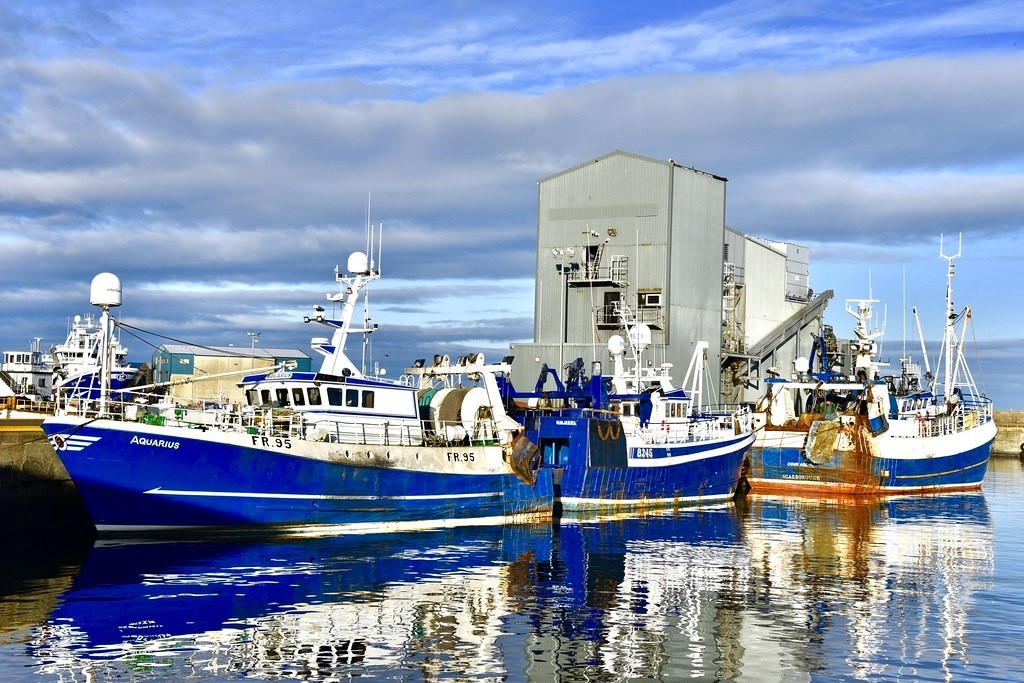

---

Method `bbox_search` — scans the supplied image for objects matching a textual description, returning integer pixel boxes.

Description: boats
[52,312,140,403]
[37,186,556,549]
[500,224,766,526]
[740,229,1002,496]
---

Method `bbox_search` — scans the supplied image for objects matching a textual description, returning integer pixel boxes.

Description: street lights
[246,332,260,374]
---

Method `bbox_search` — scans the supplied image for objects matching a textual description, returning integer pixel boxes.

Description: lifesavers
[197,400,201,405]
[661,420,670,434]
